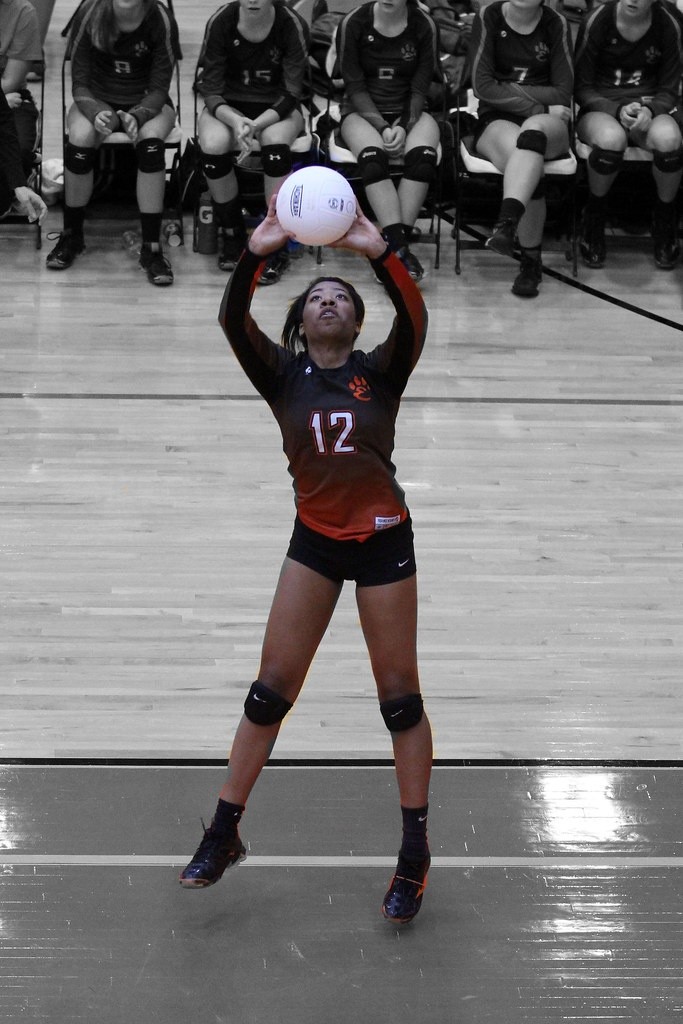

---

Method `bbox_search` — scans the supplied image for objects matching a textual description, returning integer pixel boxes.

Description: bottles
[196,192,219,255]
[122,230,142,258]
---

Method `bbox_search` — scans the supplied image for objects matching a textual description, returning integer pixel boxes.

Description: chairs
[0,0,683,277]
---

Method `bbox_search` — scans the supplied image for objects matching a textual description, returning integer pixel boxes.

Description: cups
[162,222,181,247]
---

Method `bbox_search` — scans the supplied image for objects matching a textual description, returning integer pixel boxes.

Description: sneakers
[582,208,605,267]
[257,256,290,283]
[488,218,518,256]
[138,250,173,284]
[179,830,247,889]
[44,230,86,269]
[654,221,680,266]
[512,261,542,298]
[218,240,246,270]
[383,853,433,925]
[401,252,423,281]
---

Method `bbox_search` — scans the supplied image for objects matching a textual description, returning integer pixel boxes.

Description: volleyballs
[274,164,358,248]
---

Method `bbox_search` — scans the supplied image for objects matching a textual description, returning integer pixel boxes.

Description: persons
[180,192,431,923]
[0,0,683,297]
